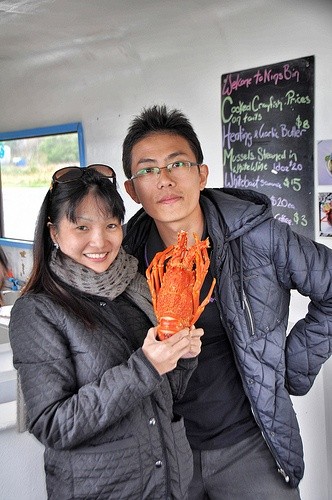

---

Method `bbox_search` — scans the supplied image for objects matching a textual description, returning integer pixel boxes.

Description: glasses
[49,164,116,196]
[130,160,200,182]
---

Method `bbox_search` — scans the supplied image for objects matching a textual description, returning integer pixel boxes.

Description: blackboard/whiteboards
[221,54,316,243]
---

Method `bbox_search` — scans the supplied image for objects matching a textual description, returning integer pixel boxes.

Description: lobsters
[145,229,217,342]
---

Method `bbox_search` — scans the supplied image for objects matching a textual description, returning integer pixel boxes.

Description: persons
[0,247,11,306]
[10,167,204,500]
[121,108,332,500]
